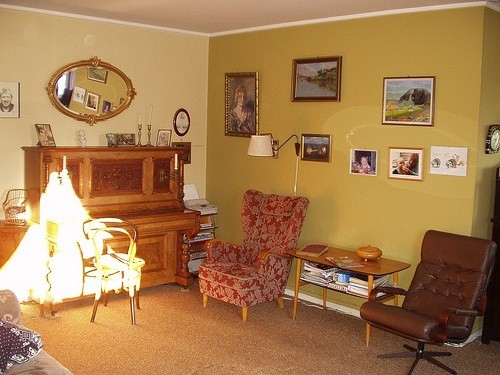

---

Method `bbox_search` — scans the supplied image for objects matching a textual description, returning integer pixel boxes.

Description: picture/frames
[0,82,20,118]
[300,134,332,163]
[429,145,468,176]
[349,150,378,176]
[35,123,56,147]
[388,147,424,182]
[157,129,172,148]
[85,92,99,112]
[224,71,259,137]
[101,99,113,113]
[381,75,436,126]
[72,87,87,103]
[291,55,342,102]
[87,68,107,83]
[173,108,191,136]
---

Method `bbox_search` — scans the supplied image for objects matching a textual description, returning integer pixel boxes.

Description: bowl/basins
[357,245,383,263]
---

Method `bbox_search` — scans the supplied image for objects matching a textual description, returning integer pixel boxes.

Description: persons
[78,129,87,146]
[393,153,419,175]
[355,151,370,169]
[0,88,14,112]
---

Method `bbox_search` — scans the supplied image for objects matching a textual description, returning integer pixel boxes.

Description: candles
[149,104,153,125]
[174,154,179,168]
[63,156,67,169]
[139,112,141,124]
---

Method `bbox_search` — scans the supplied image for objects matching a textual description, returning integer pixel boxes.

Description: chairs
[82,218,146,325]
[198,189,309,322]
[360,230,496,375]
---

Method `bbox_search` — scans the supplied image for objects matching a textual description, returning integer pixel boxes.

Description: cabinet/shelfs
[185,209,215,276]
[285,246,412,347]
[0,146,201,316]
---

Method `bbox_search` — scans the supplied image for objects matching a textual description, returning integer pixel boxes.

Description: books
[183,223,214,243]
[300,261,388,296]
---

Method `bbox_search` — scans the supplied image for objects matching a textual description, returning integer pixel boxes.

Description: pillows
[0,320,45,375]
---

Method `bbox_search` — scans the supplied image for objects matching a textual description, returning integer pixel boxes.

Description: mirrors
[46,56,138,125]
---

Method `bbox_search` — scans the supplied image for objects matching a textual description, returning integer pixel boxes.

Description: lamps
[247,132,300,158]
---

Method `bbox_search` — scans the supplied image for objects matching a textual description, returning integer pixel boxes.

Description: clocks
[485,125,500,154]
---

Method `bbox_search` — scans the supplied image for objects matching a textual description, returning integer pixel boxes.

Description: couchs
[0,289,74,375]
